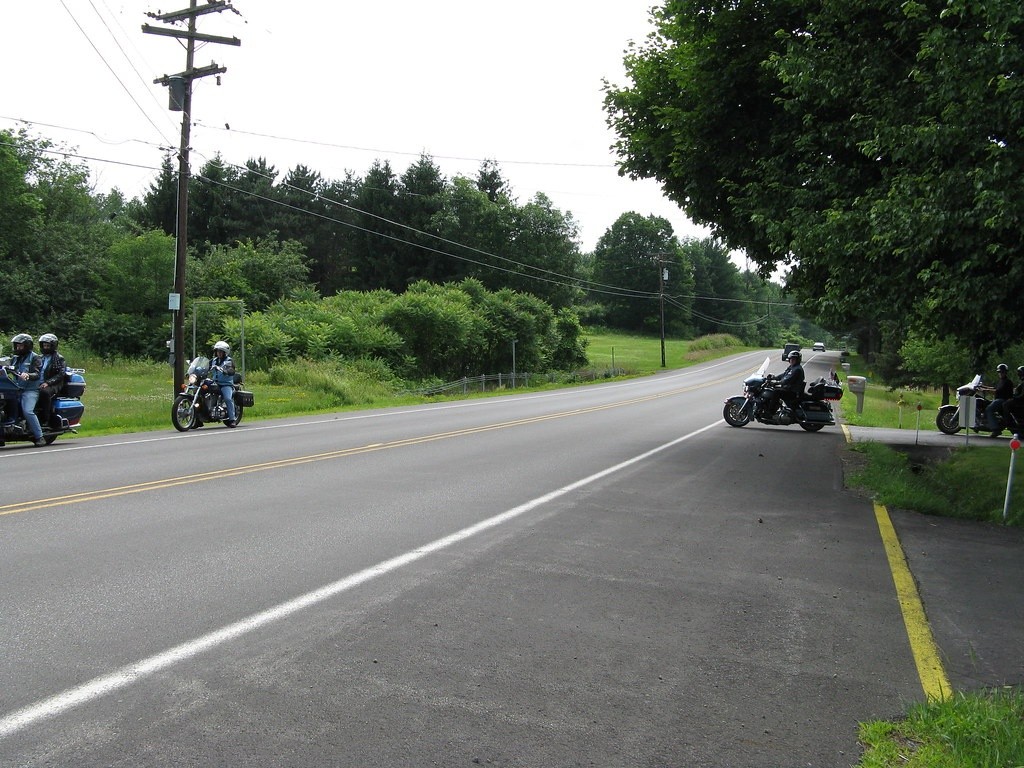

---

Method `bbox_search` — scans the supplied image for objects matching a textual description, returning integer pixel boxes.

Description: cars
[813,342,825,352]
[782,344,802,361]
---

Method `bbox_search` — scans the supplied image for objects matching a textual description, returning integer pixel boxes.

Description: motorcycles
[0,355,86,445]
[172,356,254,432]
[936,374,1024,443]
[723,357,844,432]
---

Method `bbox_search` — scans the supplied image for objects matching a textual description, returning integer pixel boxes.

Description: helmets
[214,341,230,357]
[39,333,59,353]
[997,363,1009,374]
[11,334,33,353]
[1017,366,1024,375]
[787,351,802,362]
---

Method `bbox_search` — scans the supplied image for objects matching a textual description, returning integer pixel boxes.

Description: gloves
[20,373,29,381]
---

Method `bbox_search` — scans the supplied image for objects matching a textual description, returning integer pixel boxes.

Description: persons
[761,351,804,420]
[39,333,66,427]
[189,341,237,429]
[978,364,1024,439]
[0,333,46,446]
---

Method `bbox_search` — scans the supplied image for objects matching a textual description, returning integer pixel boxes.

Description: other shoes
[36,437,46,447]
[990,430,1001,438]
[230,420,236,428]
[760,412,773,420]
